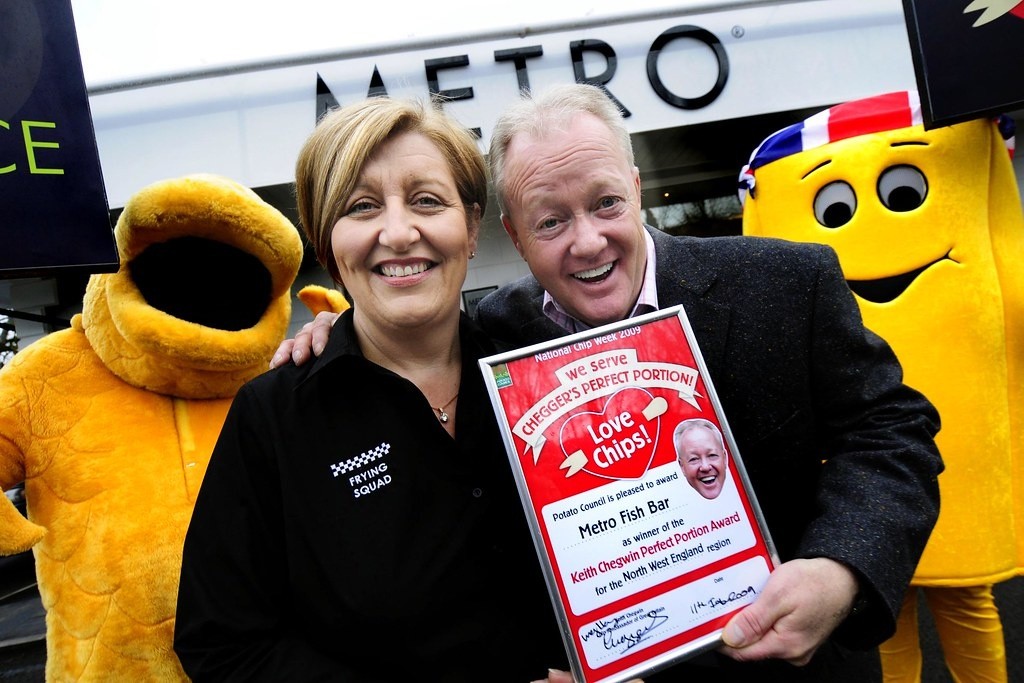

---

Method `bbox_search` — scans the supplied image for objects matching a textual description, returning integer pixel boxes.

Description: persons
[269,83,946,683]
[674,419,727,500]
[172,95,646,683]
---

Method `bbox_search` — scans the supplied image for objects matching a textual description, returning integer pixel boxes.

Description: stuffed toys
[738,91,1024,683]
[0,174,351,683]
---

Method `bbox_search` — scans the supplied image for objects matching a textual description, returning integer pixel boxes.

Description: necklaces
[432,394,458,424]
